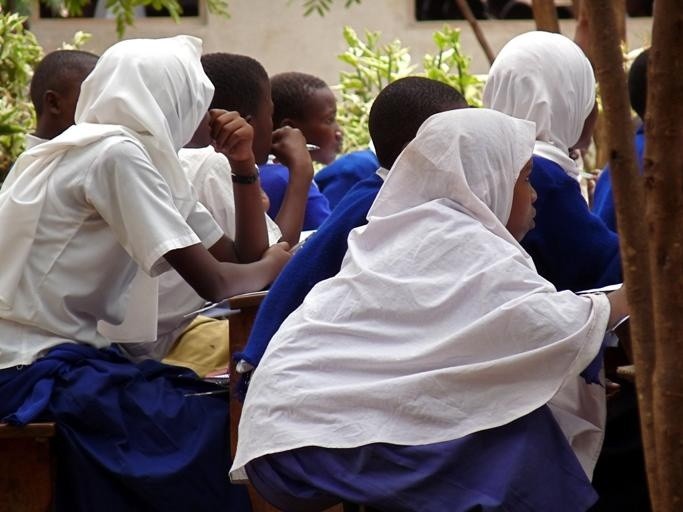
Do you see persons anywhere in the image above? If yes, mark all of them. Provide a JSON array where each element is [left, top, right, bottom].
[[1, 30, 633, 512], [594, 47, 650, 233]]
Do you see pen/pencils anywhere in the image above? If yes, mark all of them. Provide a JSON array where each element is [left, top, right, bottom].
[[288, 232, 316, 254], [305, 144, 320, 150]]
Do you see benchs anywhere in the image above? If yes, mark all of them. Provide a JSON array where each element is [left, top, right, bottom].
[[0, 420, 57, 512], [228, 289, 270, 510]]
